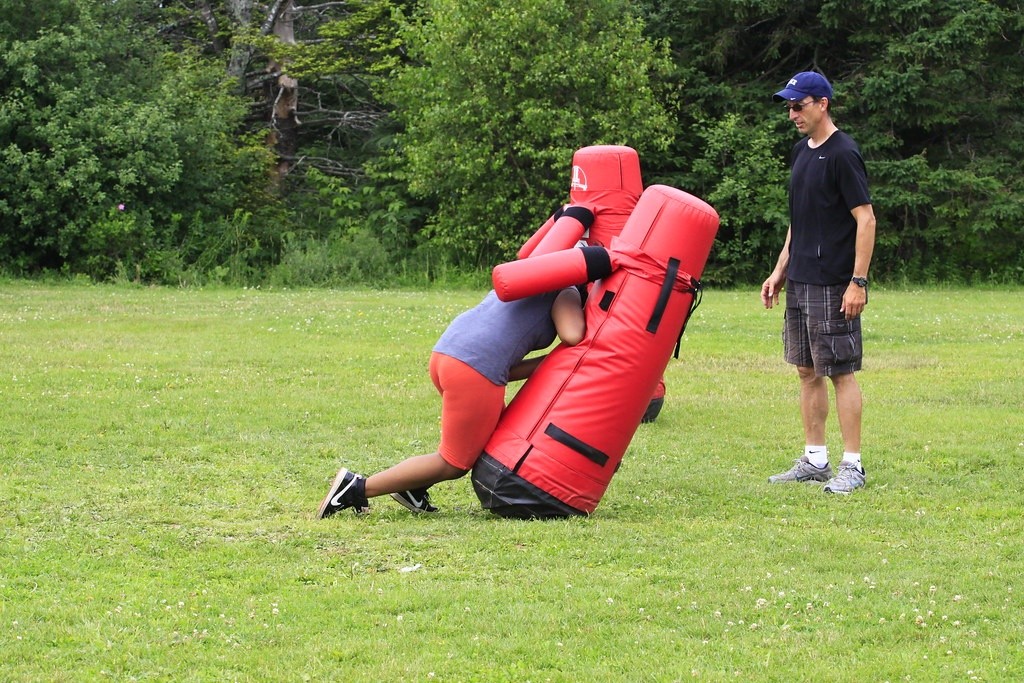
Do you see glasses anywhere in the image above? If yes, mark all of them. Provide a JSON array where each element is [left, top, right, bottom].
[[783, 100, 819, 112]]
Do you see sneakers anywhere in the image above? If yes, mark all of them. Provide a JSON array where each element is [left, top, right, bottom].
[[824, 460, 866, 495], [768, 455, 833, 484], [317, 468, 368, 520], [389, 489, 439, 515]]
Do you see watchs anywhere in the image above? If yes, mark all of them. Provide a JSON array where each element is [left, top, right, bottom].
[[852, 277, 868, 287]]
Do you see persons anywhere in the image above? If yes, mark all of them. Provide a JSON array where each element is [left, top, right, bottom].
[[319, 243, 585, 519], [760, 71, 877, 494]]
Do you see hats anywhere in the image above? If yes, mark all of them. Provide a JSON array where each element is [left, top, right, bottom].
[[772, 72, 832, 102]]
